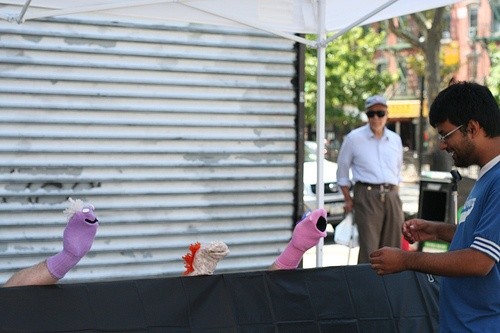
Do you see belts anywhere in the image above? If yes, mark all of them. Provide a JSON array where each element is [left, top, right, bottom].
[[356, 180, 398, 191]]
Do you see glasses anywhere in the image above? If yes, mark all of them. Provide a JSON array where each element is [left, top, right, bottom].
[[438, 124, 464, 139], [365, 110, 386, 118]]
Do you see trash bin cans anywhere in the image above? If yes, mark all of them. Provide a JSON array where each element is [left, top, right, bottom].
[[418, 178, 455, 224]]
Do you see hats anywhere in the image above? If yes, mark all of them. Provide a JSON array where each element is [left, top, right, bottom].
[[365, 95, 389, 107]]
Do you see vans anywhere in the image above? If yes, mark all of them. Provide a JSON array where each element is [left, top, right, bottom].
[[303, 141, 360, 223]]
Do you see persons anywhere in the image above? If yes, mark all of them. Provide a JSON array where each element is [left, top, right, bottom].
[[336, 95, 403, 264], [268, 208, 327, 269], [0, 207, 98, 286], [368, 81, 500, 333], [186, 241, 228, 276]]
